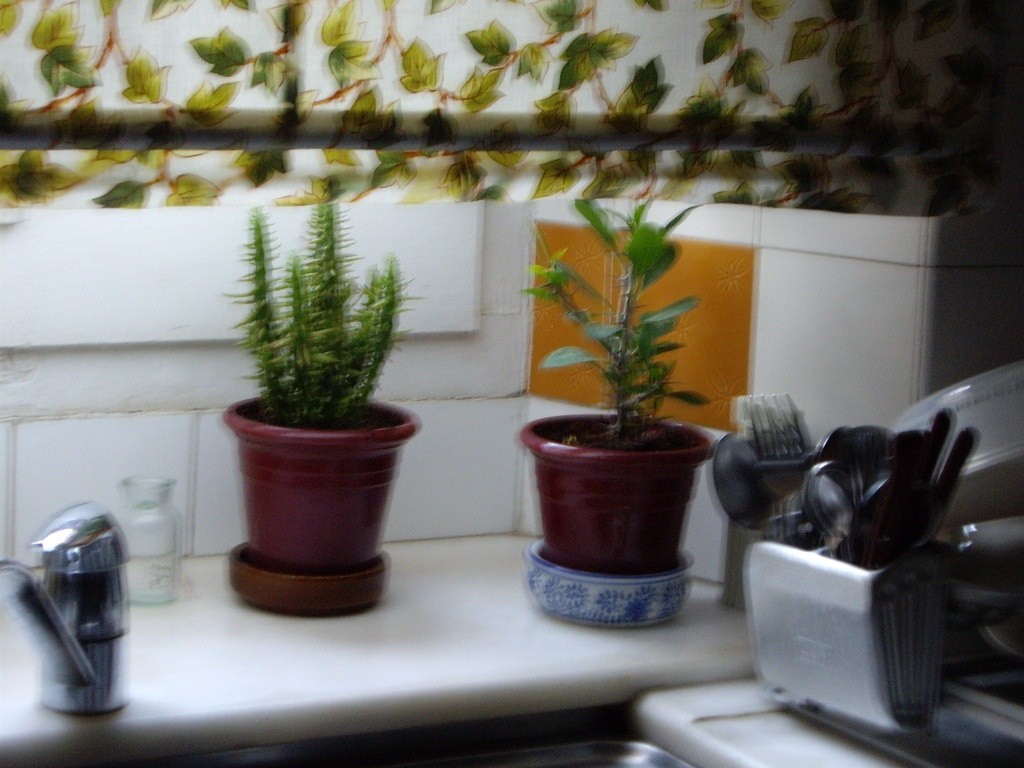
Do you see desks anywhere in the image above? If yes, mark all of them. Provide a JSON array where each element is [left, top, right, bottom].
[[636, 677, 901, 768], [0, 536, 752, 768]]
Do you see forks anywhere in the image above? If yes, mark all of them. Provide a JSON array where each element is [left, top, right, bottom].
[[737, 393, 808, 543]]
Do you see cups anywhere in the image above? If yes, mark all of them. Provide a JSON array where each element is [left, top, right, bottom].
[[720, 518, 770, 612]]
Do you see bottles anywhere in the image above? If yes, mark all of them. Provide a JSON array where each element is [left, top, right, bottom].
[[120, 477, 182, 604]]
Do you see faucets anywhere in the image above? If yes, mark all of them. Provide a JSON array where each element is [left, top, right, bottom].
[[0, 498, 133, 717]]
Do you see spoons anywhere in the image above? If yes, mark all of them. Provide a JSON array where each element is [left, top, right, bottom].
[[803, 425, 896, 569]]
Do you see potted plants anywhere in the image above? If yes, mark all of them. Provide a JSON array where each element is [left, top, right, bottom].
[[520, 182, 714, 578], [222, 178, 423, 572]]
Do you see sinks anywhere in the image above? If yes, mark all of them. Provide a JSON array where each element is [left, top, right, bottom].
[[0, 737, 701, 767]]
